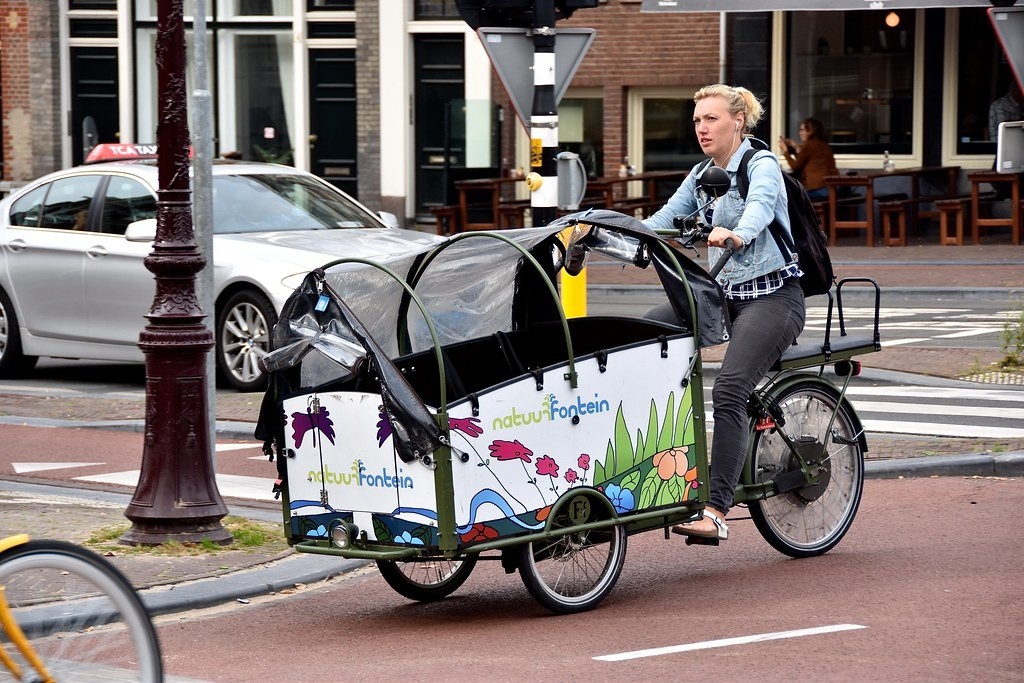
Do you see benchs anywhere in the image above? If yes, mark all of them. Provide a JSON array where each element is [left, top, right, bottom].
[[497, 199, 607, 230], [429, 198, 531, 237], [608, 200, 671, 217], [935, 192, 1007, 246], [557, 196, 650, 222], [812, 192, 907, 247], [878, 194, 967, 248]]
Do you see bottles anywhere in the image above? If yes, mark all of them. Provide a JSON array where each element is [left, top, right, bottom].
[[883, 151, 894, 172]]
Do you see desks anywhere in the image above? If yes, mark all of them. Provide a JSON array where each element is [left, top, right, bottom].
[[822, 166, 961, 248], [599, 170, 691, 220], [968, 170, 1024, 245], [454, 175, 529, 232]]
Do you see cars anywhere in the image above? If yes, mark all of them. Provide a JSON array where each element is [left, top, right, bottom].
[[0, 142, 453, 394]]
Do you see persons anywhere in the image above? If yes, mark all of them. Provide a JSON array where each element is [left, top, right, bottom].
[[779, 117, 842, 202], [989, 77, 1024, 142], [603, 82, 807, 541]]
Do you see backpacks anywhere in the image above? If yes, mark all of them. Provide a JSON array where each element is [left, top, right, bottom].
[[696, 149, 835, 299]]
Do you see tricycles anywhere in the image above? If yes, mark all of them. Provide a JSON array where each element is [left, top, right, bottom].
[[256, 166, 883, 613]]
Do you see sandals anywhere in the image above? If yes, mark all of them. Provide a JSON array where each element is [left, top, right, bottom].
[[672, 509, 730, 540]]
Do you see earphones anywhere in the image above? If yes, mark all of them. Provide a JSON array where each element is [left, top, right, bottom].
[[735, 121, 739, 131]]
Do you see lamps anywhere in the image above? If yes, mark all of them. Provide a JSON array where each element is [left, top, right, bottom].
[[885, 9, 900, 26]]
